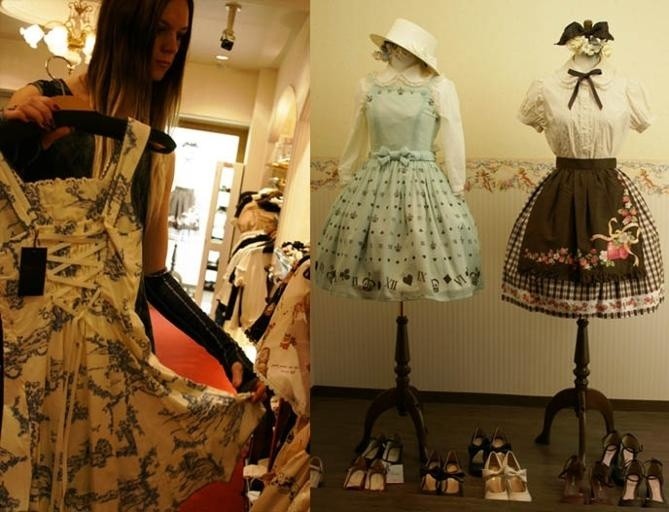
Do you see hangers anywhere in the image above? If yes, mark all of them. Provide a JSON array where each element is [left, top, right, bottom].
[[1, 56, 176, 165]]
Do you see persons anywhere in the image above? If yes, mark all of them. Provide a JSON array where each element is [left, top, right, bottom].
[[0, 0, 267, 404], [502, 19, 667, 318], [314, 19, 483, 302]]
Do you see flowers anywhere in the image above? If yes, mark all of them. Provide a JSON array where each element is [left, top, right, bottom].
[[555, 20, 613, 58]]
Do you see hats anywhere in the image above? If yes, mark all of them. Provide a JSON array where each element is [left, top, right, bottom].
[[370, 18, 441, 76]]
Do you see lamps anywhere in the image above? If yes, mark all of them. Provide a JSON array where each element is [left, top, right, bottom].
[[17, 1, 97, 67]]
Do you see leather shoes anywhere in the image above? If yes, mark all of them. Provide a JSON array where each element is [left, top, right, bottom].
[[310, 425, 666, 507]]
